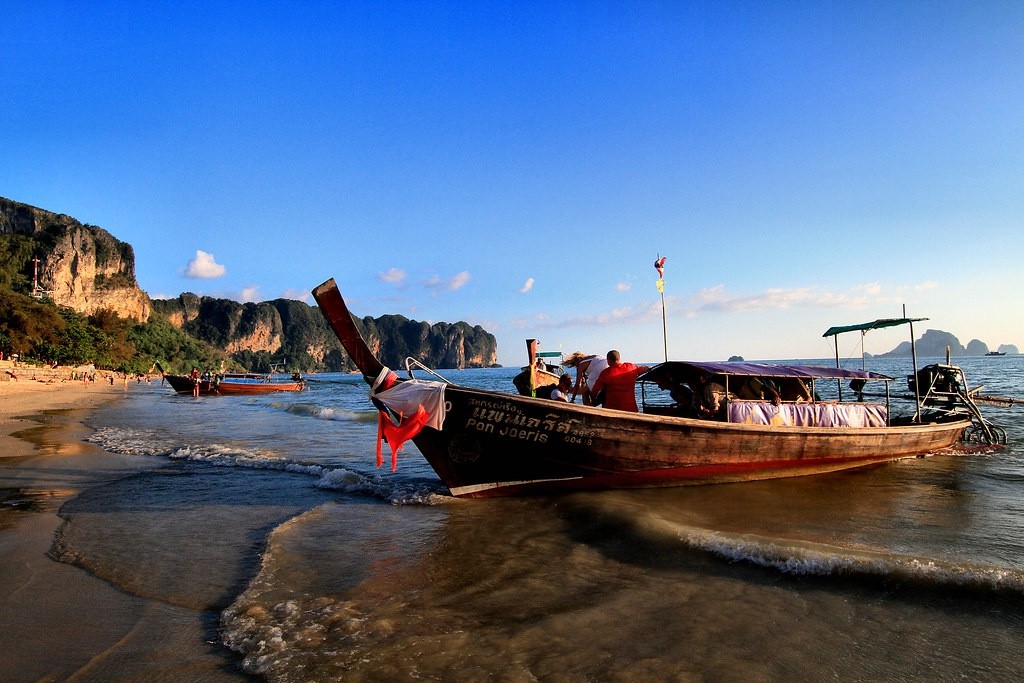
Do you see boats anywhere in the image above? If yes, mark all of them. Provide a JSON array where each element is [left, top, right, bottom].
[[312, 277, 975, 496], [985, 351, 1007, 355], [155, 361, 305, 396]]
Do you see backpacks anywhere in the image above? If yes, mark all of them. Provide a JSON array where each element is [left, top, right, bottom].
[[748, 374, 778, 400]]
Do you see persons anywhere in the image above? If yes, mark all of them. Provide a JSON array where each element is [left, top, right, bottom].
[[12, 371, 18, 382], [592, 350, 649, 413], [0, 351, 3, 360], [191, 367, 221, 397], [104, 373, 114, 386], [136, 377, 141, 385], [560, 351, 609, 408], [12, 357, 18, 367], [122, 373, 128, 387], [551, 374, 573, 403], [60, 362, 63, 366], [537, 358, 546, 371], [71, 371, 97, 383], [654, 370, 812, 421]]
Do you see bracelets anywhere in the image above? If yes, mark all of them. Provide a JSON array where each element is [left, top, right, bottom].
[[571, 398, 575, 400]]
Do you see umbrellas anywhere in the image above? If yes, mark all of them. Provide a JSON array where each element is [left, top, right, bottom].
[[12, 354, 18, 357]]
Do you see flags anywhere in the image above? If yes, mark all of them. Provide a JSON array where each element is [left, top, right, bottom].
[[536, 339, 540, 345], [654, 257, 666, 276]]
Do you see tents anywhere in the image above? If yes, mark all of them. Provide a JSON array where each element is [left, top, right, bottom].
[[73, 365, 95, 382]]
